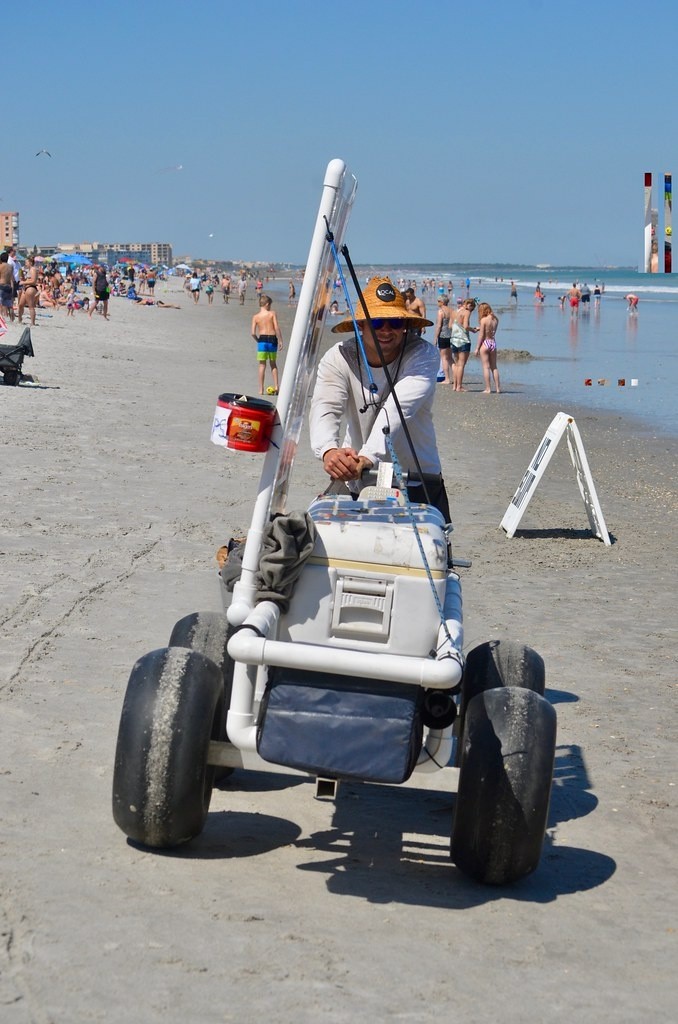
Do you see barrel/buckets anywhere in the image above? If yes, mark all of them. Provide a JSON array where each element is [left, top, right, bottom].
[[211, 393, 276, 452], [217, 570, 233, 614]]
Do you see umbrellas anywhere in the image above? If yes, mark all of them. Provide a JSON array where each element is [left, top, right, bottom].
[[0, 252, 193, 285]]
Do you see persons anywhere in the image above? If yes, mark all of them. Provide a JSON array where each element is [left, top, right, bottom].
[[173, 263, 640, 334], [433, 292, 502, 394], [0, 246, 181, 327], [309, 274, 454, 529], [250, 294, 283, 396]]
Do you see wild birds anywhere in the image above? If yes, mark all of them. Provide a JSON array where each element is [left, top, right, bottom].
[[35, 150, 52, 158]]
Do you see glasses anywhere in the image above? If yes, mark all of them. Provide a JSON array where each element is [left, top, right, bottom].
[[366, 318, 404, 330]]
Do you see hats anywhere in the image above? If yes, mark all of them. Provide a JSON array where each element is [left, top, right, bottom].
[[186, 274, 192, 277], [331, 276, 434, 332], [102, 263, 108, 269]]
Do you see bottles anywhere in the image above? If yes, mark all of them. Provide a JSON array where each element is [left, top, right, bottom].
[[19, 269, 23, 279]]
